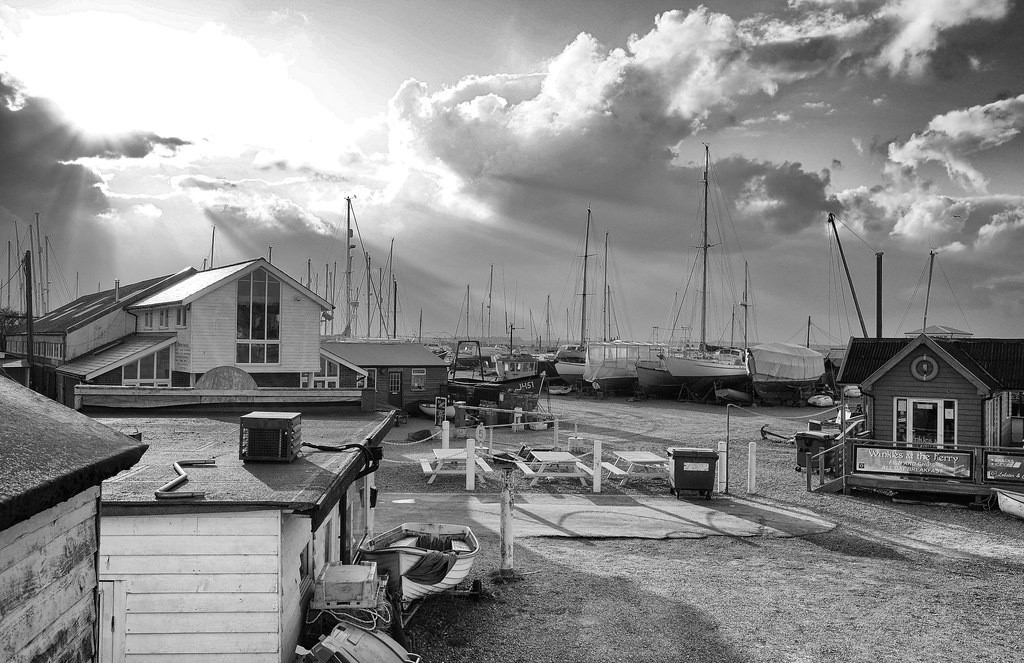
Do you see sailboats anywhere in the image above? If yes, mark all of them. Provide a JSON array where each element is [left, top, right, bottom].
[[1, 130, 975, 407]]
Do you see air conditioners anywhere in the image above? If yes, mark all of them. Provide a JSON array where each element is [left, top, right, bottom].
[[239, 410, 302, 462]]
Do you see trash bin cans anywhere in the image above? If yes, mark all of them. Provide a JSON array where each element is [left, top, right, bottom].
[[794, 429, 841, 471], [667, 447, 719, 501]]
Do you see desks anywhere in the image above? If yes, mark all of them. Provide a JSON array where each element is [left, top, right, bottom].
[[521, 451, 588, 488], [427, 449, 487, 485], [607, 451, 668, 486]]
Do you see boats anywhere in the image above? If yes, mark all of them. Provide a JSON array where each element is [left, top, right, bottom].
[[358, 521, 480, 604], [989, 487, 1024, 520], [447, 369, 546, 412]]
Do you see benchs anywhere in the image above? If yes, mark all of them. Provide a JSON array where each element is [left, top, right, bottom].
[[576, 462, 594, 476], [601, 462, 629, 476], [516, 462, 535, 477], [476, 457, 494, 474], [420, 459, 433, 476]]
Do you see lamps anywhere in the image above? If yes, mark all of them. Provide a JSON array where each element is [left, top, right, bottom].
[[923, 364, 928, 374]]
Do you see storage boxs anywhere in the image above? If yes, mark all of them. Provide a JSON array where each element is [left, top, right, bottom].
[[310, 561, 382, 608], [795, 430, 835, 474], [666, 447, 720, 499]]
